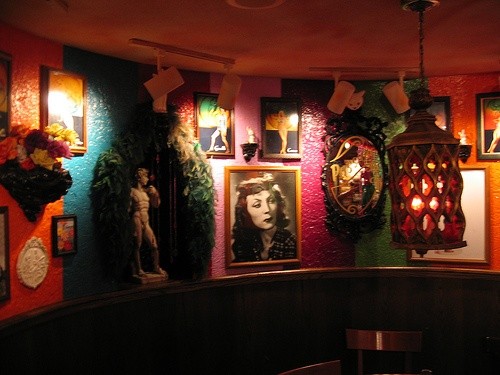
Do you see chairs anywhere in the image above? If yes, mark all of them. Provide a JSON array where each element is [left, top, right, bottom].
[[345, 327, 425, 375], [278, 359, 342, 375]]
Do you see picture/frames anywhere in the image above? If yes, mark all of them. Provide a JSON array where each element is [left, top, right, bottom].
[[51, 213, 77, 257], [405, 164, 493, 267], [1, 203, 11, 302], [258, 96, 303, 160], [222, 165, 303, 269], [191, 92, 237, 160], [406, 95, 454, 137], [90, 114, 216, 289], [0, 50, 15, 141], [39, 63, 89, 155], [475, 91, 500, 160], [322, 115, 391, 243]]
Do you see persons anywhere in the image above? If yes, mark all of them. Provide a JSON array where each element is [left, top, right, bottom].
[[127, 166, 167, 277], [231, 172, 298, 262]]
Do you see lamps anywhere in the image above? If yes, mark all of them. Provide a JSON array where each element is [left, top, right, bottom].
[[144, 55, 185, 101], [217, 63, 242, 110], [383, 72, 410, 114], [387, 87, 467, 257], [325, 71, 356, 115]]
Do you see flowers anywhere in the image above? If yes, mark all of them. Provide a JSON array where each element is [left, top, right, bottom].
[[9, 122, 85, 174]]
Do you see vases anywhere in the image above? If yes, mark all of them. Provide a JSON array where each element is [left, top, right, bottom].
[[1, 156, 73, 224]]
